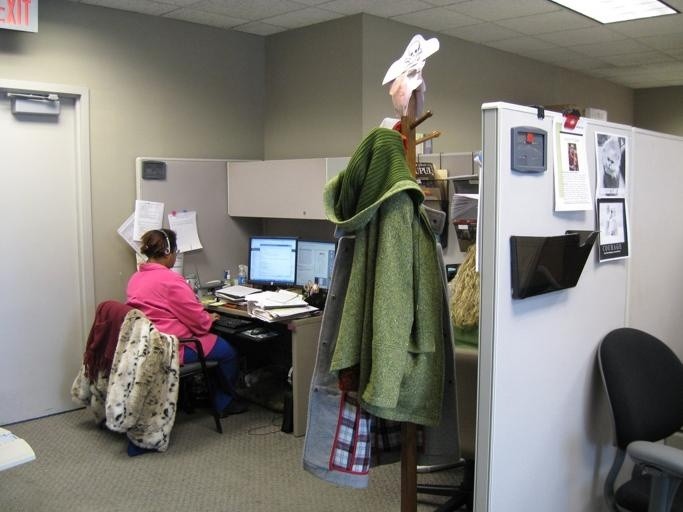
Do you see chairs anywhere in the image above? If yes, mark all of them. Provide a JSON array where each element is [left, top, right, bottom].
[[94, 301, 224, 434], [598, 327, 683, 512]]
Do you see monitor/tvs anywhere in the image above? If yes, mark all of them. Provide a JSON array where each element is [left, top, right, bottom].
[[295, 239, 338, 291], [246, 234, 298, 286]]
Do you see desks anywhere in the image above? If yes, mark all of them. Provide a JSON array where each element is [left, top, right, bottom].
[[197, 303, 326, 435]]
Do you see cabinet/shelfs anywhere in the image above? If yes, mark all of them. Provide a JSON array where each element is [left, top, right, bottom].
[[415, 153, 478, 451]]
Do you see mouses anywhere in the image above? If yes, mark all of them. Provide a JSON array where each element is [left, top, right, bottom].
[[251, 327, 267, 334]]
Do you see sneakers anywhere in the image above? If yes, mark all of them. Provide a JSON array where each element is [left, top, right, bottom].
[[218, 401, 250, 418]]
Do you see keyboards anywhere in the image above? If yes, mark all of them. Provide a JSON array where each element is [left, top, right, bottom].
[[215, 316, 252, 329]]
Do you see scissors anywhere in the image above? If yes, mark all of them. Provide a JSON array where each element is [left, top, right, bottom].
[[304, 280, 312, 293]]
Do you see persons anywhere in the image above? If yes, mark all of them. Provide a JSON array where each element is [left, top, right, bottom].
[[123, 228, 251, 419]]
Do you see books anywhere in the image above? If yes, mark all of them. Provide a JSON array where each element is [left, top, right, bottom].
[[414, 160, 448, 239], [212, 282, 320, 323], [520, 232, 582, 299], [449, 193, 478, 222]]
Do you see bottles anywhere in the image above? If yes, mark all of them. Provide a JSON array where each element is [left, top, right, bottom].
[[312, 276, 319, 293], [238, 267, 246, 286]]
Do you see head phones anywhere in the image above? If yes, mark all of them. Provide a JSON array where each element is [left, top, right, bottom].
[[144, 228, 172, 255]]
